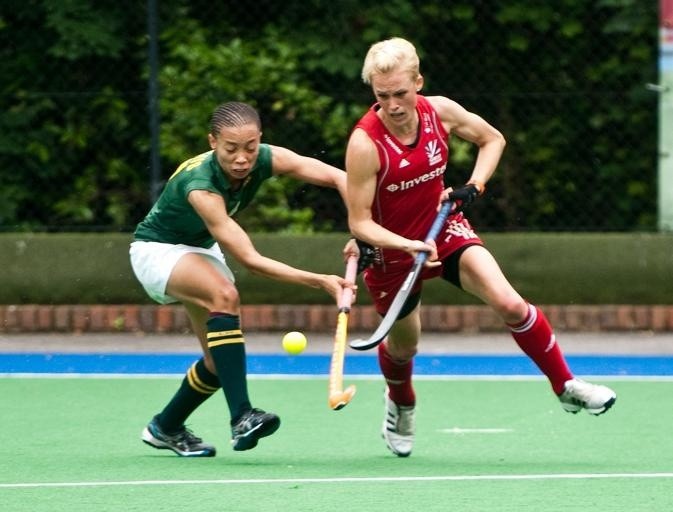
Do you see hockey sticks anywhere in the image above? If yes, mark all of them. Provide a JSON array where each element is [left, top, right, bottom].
[[349, 199, 454, 350], [327, 254, 357, 410]]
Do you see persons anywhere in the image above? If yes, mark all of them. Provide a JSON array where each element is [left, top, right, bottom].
[[129, 100, 377, 459], [343, 37, 621, 458]]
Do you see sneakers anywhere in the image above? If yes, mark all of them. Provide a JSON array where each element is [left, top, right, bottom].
[[141, 415, 216, 458], [384, 387, 414, 457], [231, 409, 280, 451], [558, 376, 617, 417]]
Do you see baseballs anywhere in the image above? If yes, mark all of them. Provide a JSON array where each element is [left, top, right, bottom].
[[283, 332, 307, 355]]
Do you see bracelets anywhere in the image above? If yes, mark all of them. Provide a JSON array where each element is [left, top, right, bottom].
[[464, 179, 486, 197]]
